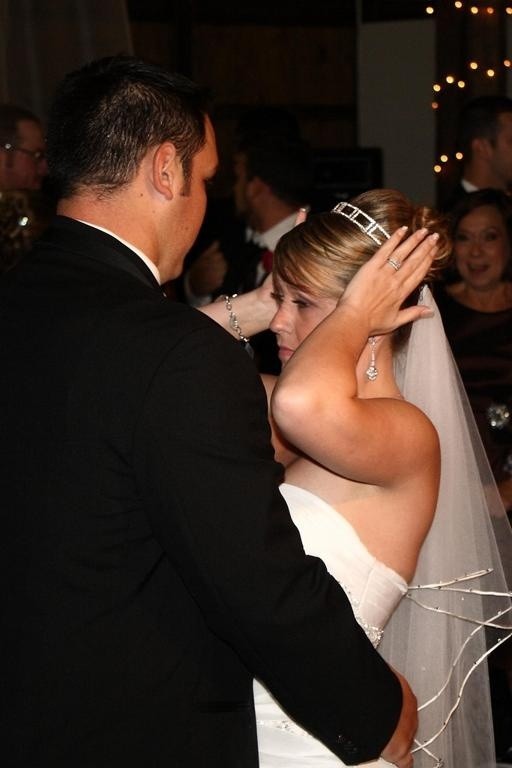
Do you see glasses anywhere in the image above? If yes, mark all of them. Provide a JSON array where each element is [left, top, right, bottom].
[[13, 143, 46, 159]]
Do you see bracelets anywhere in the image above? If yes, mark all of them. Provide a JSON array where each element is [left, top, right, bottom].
[[221, 294, 250, 344]]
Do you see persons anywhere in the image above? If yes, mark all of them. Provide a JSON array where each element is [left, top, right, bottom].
[[428, 185, 508, 535], [160, 93, 510, 316], [2, 108, 46, 229], [188, 181, 445, 768], [4, 50, 420, 768]]
